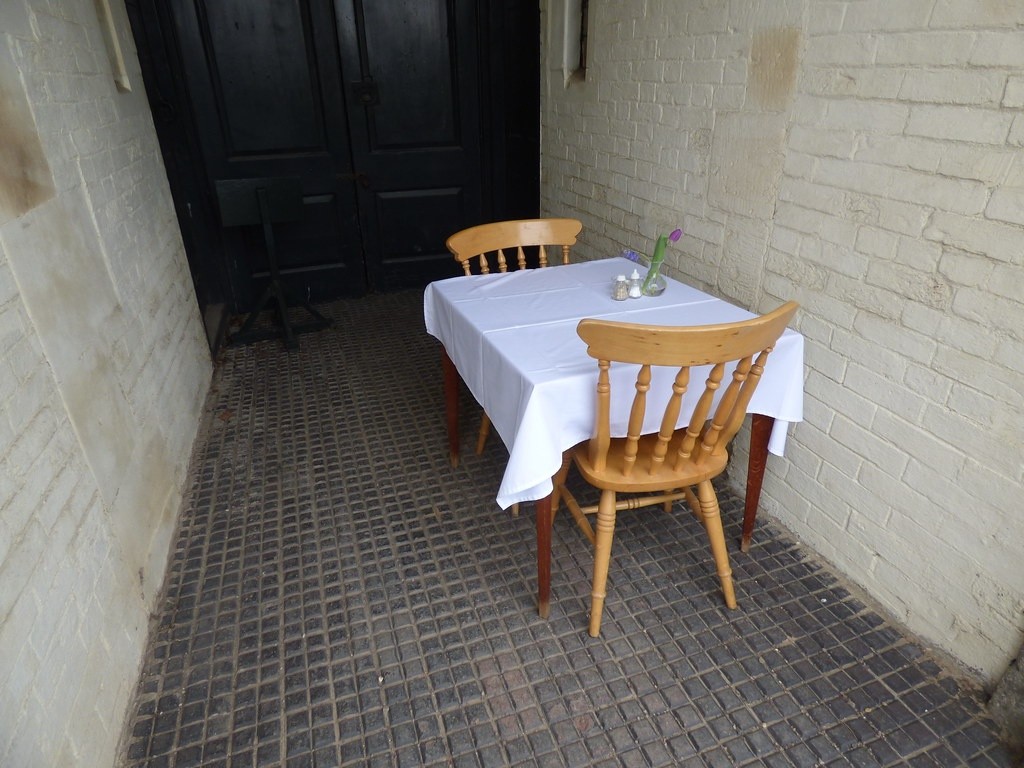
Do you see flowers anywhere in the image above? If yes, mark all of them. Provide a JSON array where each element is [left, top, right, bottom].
[[623, 229, 683, 294]]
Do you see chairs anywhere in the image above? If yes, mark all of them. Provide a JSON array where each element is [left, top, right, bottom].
[[552, 300, 800, 638], [445, 217, 585, 520]]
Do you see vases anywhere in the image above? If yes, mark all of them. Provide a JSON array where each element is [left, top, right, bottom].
[[639, 258, 667, 296]]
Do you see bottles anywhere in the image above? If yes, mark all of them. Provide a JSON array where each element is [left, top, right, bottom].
[[612, 269, 642, 301]]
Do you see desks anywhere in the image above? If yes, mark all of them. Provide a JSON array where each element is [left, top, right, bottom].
[[421, 256, 805, 618]]
